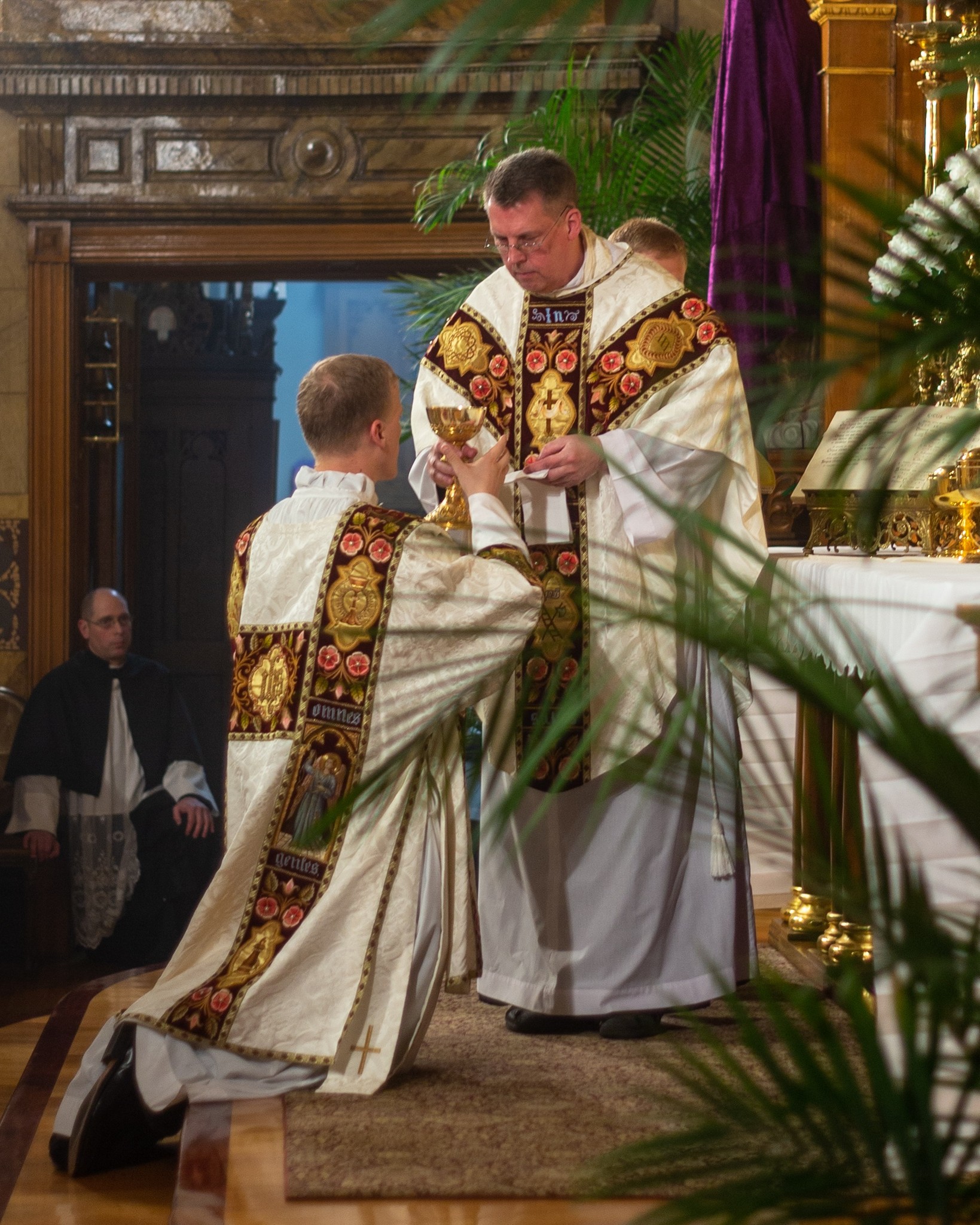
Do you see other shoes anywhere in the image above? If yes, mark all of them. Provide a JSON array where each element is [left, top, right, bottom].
[[600, 1010, 662, 1041], [505, 1005, 600, 1035]]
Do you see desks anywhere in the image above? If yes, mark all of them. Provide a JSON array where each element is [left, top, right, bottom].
[[734, 553, 980, 952]]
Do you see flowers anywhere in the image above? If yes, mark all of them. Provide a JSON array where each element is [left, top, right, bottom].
[[869, 143, 980, 303]]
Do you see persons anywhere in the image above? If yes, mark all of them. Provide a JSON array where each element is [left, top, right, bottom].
[[404, 147, 766, 1041], [48, 353, 544, 1186], [607, 218, 691, 290], [5, 586, 224, 998]]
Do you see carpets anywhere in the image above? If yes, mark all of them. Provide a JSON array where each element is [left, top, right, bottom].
[[283, 910, 907, 1202]]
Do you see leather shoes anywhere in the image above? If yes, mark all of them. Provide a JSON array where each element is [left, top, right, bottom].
[[48, 1018, 184, 1178]]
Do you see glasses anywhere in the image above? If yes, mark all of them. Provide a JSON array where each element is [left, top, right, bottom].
[[85, 613, 130, 628], [484, 205, 571, 254]]
